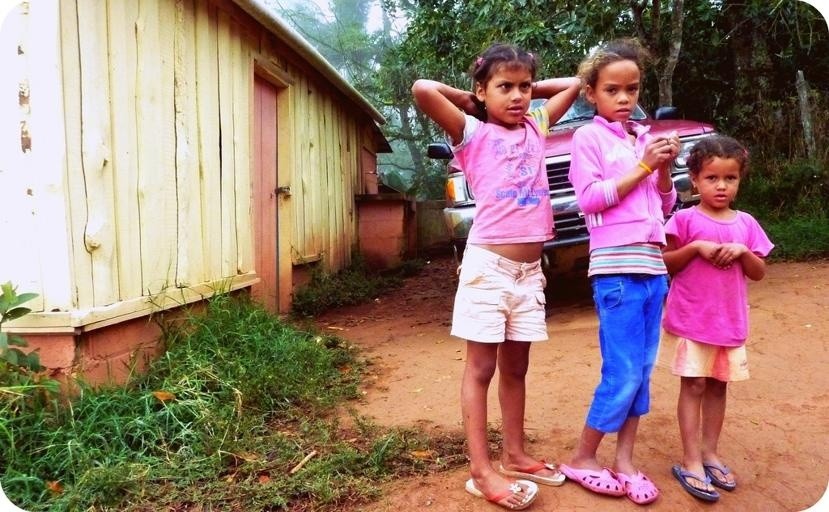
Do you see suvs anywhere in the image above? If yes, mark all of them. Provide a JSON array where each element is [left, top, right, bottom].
[[426, 83, 716, 295]]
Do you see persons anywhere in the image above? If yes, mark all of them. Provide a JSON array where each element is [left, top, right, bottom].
[[557, 36, 682, 504], [663, 136, 776, 502], [412, 44, 581, 508]]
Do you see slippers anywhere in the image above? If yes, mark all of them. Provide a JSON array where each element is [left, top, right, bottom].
[[464, 476, 540, 511], [703, 460, 736, 491], [498, 460, 566, 486], [604, 463, 659, 505], [672, 463, 720, 502], [559, 462, 625, 497]]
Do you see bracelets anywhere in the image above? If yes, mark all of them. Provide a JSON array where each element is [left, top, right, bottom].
[[638, 159, 654, 175]]
